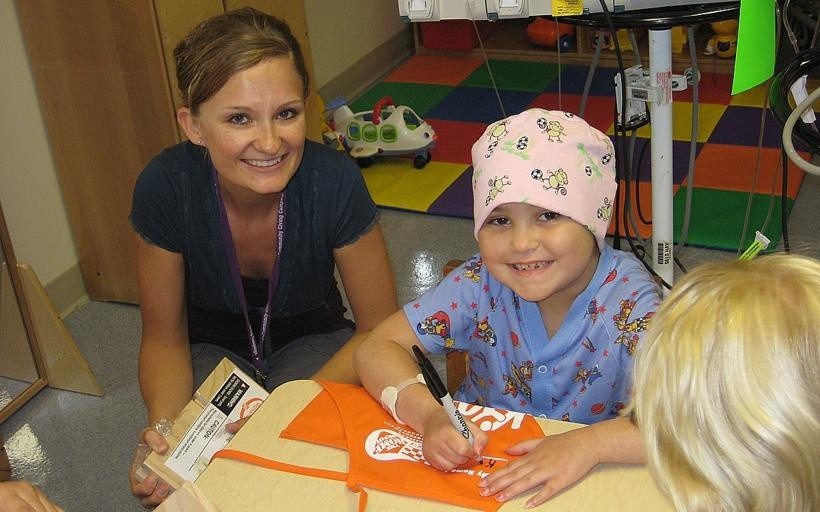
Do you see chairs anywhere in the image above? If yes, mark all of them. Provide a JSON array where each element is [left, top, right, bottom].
[[442, 260, 469, 397]]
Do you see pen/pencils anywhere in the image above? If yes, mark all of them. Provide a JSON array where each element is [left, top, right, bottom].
[[412, 345, 484, 465]]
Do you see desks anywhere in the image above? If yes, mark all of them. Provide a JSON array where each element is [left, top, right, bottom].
[[151, 379, 678, 512]]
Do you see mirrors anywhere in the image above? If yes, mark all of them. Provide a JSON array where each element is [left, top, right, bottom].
[[0, 202, 105, 426]]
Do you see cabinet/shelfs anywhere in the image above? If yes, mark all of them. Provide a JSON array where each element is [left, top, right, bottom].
[[412, 21, 735, 74]]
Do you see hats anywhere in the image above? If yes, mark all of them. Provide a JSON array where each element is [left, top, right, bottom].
[[471, 107, 618, 253]]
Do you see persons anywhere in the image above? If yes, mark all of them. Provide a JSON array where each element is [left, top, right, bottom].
[[124, 5, 404, 508], [0, 475, 65, 512], [358, 102, 668, 511], [624, 248, 820, 511]]
